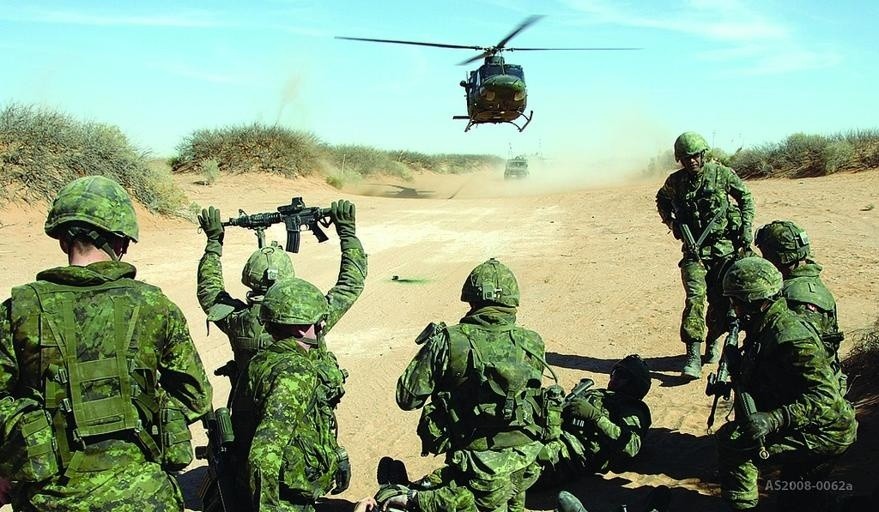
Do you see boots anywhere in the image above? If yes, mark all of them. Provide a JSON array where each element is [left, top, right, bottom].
[[682, 340, 702, 380], [705, 331, 720, 363]]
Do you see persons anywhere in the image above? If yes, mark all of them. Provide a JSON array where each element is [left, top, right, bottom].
[[705, 257, 861, 510], [377, 354, 652, 500], [1, 176, 212, 510], [196, 200, 367, 402], [755, 219, 850, 400], [394, 257, 563, 511], [354, 480, 476, 510], [228, 277, 340, 511], [655, 133, 755, 379]]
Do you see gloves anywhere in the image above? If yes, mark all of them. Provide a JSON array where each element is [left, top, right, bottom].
[[738, 223, 753, 248], [197, 205, 225, 256], [570, 398, 602, 420], [331, 199, 364, 251], [742, 410, 785, 441], [332, 459, 351, 495]]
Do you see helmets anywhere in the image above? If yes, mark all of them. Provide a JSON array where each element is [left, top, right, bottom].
[[460, 259, 520, 306], [44, 175, 138, 243], [675, 131, 711, 163], [259, 277, 330, 325], [241, 246, 295, 292], [723, 257, 784, 301], [610, 354, 651, 389], [759, 220, 811, 264]]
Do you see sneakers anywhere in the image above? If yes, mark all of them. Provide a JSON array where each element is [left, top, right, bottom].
[[378, 457, 391, 489], [393, 461, 408, 488]]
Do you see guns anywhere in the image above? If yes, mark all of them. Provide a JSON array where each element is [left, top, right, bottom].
[[671, 200, 704, 267], [722, 345, 770, 460], [196, 360, 235, 512], [706, 304, 741, 435], [198, 197, 332, 253]]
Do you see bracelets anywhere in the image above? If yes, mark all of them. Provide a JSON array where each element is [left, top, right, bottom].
[[406, 488, 420, 510]]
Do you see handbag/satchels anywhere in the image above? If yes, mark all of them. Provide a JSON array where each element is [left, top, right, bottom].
[[159, 396, 195, 470], [0, 397, 59, 485]]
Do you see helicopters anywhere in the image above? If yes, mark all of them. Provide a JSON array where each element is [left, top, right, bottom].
[[335, 14, 643, 134]]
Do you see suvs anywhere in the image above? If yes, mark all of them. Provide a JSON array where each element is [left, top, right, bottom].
[[502, 156, 529, 179]]
